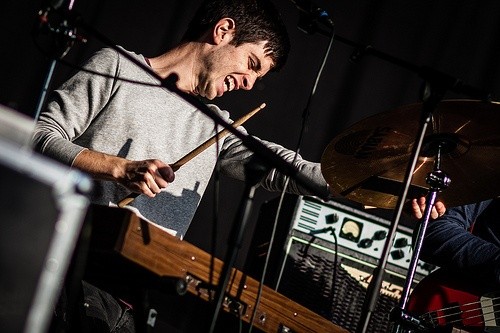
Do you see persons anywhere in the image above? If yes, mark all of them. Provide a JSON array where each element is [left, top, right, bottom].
[[416, 162, 500, 290], [34, 1, 445, 333]]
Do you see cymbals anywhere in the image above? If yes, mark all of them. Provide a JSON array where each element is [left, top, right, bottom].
[[320, 99, 500, 210]]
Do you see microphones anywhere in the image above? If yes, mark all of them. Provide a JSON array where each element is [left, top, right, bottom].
[[290, 0, 335, 28]]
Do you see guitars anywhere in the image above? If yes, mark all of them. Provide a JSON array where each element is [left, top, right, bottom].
[[403, 255, 499, 333]]
